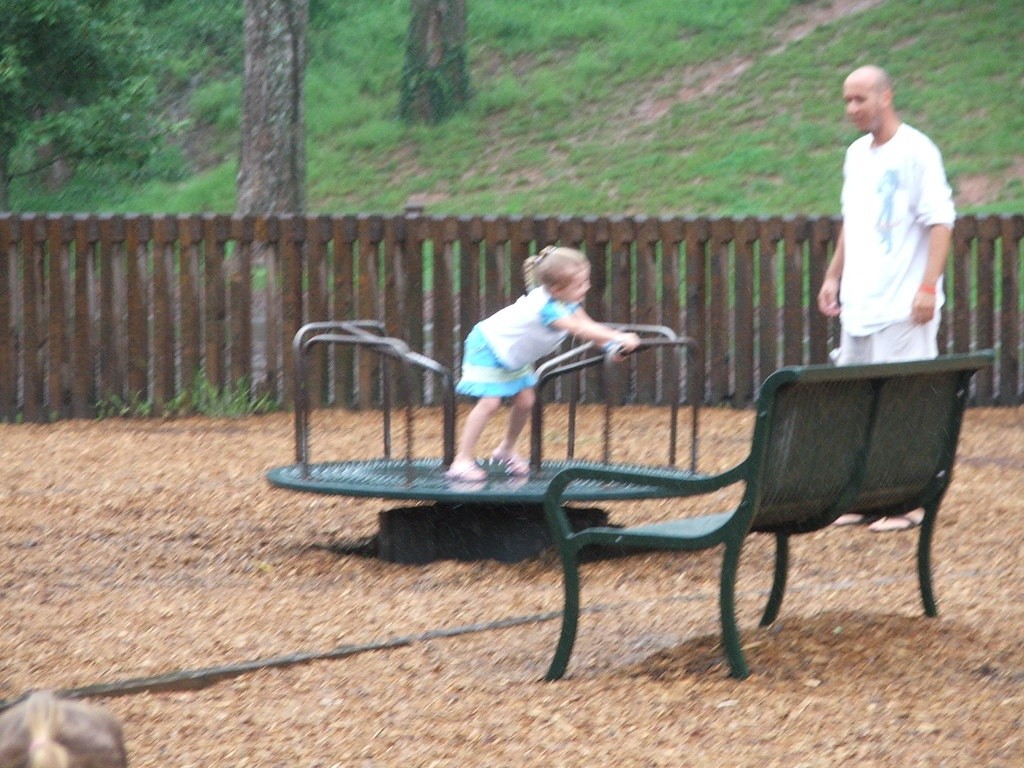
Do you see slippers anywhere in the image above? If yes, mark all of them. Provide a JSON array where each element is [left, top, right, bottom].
[[872, 514, 922, 532], [834, 512, 878, 526]]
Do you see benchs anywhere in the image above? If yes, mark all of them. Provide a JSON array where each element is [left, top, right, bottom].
[[544, 349, 997, 689]]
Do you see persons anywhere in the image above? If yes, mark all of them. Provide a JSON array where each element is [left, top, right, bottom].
[[817, 65, 957, 532], [446, 246, 641, 483]]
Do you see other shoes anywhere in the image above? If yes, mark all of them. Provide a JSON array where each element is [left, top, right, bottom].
[[493, 449, 528, 476], [449, 457, 486, 480]]
